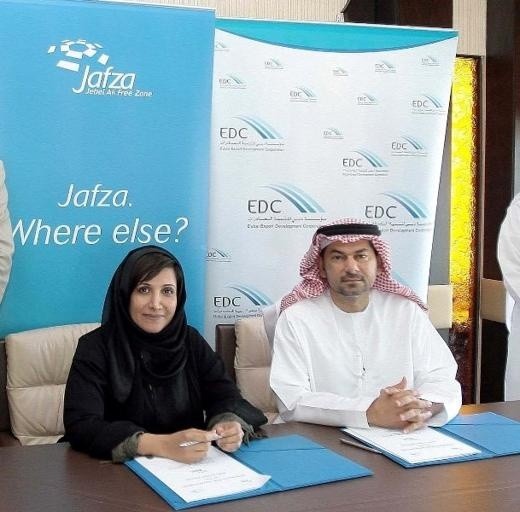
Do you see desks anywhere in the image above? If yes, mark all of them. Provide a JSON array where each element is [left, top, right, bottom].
[[0, 400, 518, 511]]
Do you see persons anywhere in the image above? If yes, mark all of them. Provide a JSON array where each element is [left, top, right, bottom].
[[0, 160, 16, 304], [62, 244, 270, 466], [270, 216, 464, 433], [495, 191, 520, 402]]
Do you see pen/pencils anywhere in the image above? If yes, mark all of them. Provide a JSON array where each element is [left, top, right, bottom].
[[178, 436, 220, 448], [340, 439, 383, 455]]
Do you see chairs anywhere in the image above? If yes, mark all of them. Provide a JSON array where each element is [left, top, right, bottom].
[[214, 315, 276, 416], [0, 323, 102, 445]]
